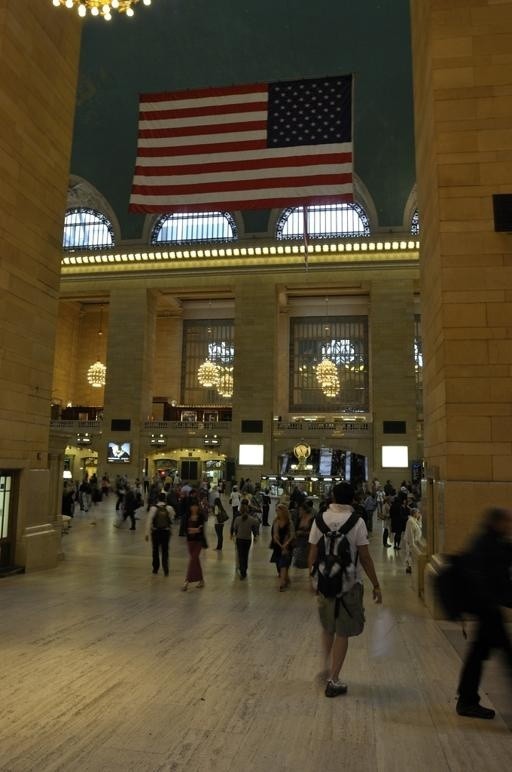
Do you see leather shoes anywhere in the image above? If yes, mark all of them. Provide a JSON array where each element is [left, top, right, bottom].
[[280, 578, 292, 593], [196, 582, 205, 588], [180, 587, 188, 591]]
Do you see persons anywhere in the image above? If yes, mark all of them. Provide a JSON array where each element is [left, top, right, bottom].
[[353, 477, 423, 575], [108, 442, 130, 458], [64, 471, 315, 592], [456, 505, 512, 718], [307, 483, 383, 698]]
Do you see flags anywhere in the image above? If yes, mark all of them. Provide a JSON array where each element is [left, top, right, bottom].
[[128, 73, 354, 214]]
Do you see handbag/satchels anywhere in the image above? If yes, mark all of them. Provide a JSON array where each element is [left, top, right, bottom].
[[216, 509, 229, 524]]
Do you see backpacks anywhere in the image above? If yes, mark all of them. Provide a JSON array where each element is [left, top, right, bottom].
[[431, 555, 475, 624], [309, 510, 360, 598], [152, 502, 172, 530]]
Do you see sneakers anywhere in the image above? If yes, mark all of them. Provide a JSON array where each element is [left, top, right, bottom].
[[323, 679, 352, 700], [456, 699, 497, 721]]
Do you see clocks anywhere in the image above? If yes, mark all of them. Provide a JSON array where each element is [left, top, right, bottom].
[[293, 441, 312, 460]]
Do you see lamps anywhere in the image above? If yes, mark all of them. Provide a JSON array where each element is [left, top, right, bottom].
[[322, 377, 341, 398], [217, 372, 234, 399], [315, 297, 338, 389], [85, 302, 107, 389], [196, 300, 220, 389]]
